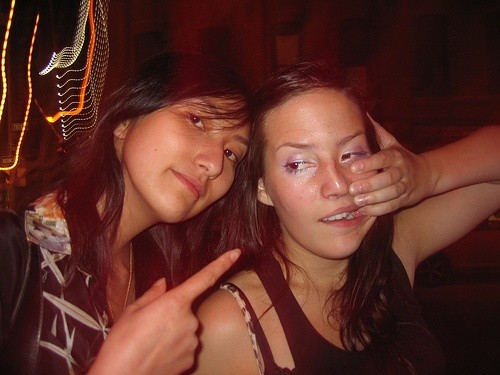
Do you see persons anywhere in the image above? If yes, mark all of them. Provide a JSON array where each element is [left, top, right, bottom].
[[196, 62, 500, 375], [0, 56, 252, 375]]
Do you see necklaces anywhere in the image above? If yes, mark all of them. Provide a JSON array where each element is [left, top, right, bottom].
[[106, 241, 132, 323]]
